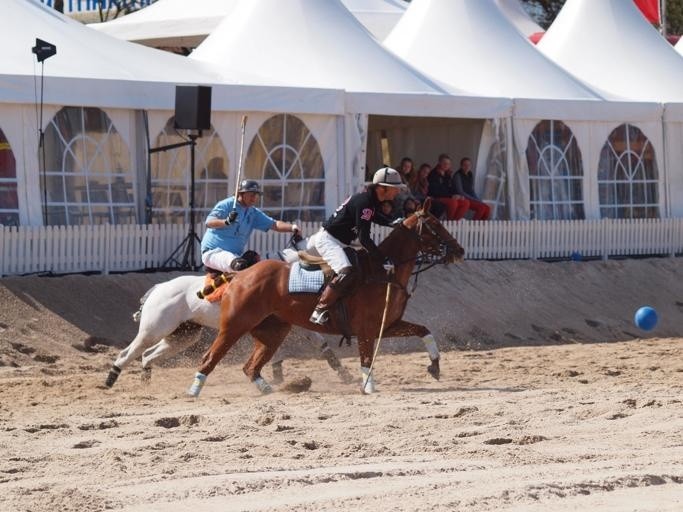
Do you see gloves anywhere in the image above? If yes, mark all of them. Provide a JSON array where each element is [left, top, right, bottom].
[[292, 224, 302, 237], [225, 211, 238, 226], [382, 256, 395, 275]]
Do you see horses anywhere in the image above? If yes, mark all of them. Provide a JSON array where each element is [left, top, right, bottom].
[[184, 196, 465, 397], [99, 238, 369, 395]]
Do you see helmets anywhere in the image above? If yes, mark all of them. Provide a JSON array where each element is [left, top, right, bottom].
[[373, 166, 408, 188], [238, 179, 264, 195]]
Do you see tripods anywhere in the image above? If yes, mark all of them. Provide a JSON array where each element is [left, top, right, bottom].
[[159, 129, 203, 271]]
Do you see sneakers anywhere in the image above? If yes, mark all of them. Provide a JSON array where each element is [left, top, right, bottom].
[[309, 308, 329, 325]]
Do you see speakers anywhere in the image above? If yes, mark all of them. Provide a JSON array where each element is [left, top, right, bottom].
[[174, 86, 211, 129]]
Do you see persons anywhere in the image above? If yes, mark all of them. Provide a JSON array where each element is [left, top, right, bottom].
[[427, 154, 470, 221], [416, 163, 447, 221], [400, 197, 417, 219], [396, 157, 422, 211], [379, 200, 403, 221], [308, 166, 406, 327], [452, 157, 490, 220], [199, 178, 302, 273]]
